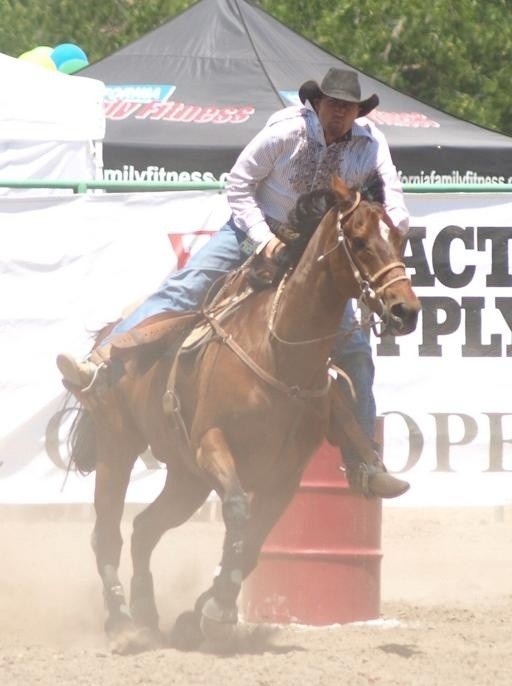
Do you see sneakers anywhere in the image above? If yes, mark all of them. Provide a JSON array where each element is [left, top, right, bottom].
[[56, 353, 107, 405], [348, 461, 410, 500]]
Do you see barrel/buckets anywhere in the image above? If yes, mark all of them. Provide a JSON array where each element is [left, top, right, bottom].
[[236, 414, 382, 625]]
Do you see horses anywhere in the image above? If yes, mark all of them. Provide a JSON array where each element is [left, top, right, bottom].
[[53, 167, 420, 655]]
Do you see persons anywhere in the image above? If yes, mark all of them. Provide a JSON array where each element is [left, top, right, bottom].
[[55, 69, 410, 500]]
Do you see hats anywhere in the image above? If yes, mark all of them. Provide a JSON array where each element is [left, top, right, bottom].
[[299, 67, 379, 118]]
